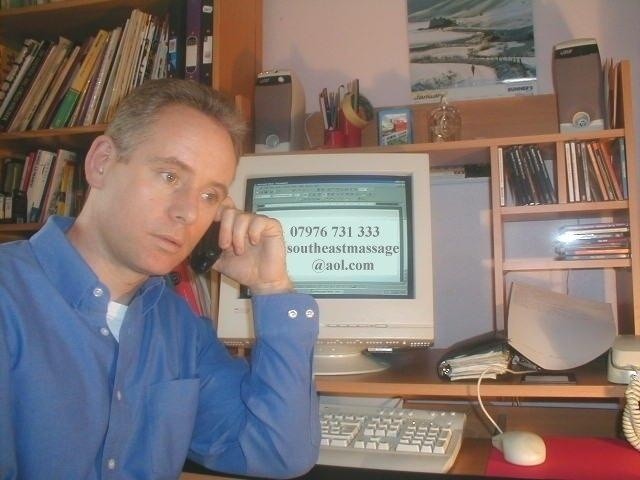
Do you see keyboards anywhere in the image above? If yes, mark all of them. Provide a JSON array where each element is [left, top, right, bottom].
[[316, 404, 466, 474]]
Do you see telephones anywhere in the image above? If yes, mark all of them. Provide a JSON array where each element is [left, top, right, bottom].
[[607, 335, 640, 384]]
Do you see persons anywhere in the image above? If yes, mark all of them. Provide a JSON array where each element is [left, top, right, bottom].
[[471, 63, 475, 76], [1, 78, 323, 477]]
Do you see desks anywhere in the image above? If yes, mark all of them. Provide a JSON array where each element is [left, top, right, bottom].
[[179, 404, 639, 479]]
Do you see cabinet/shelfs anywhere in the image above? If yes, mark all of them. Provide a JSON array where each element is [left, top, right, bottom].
[[1, 0, 264, 356], [230, 57, 640, 398]]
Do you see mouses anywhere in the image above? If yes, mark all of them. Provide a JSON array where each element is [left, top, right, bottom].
[[492, 430, 547, 466]]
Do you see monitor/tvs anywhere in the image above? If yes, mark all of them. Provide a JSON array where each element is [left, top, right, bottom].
[[216, 153, 435, 375]]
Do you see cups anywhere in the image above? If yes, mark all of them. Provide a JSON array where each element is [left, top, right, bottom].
[[323, 129, 344, 147], [341, 106, 362, 147]]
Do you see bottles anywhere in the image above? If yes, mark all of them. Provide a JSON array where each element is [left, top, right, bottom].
[[425, 92, 462, 144]]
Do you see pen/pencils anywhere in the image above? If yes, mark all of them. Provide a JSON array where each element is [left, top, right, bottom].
[[320, 79, 359, 131]]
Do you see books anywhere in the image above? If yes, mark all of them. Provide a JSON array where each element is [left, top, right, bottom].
[[498, 60, 628, 259], [436, 330, 507, 382]]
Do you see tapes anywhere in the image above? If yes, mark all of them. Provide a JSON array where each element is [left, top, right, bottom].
[[343, 94, 375, 128]]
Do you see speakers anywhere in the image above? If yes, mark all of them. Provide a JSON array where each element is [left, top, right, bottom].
[[554, 38, 606, 134], [254, 69, 305, 154]]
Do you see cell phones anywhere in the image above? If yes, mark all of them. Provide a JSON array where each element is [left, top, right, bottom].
[[190, 221, 223, 273]]
